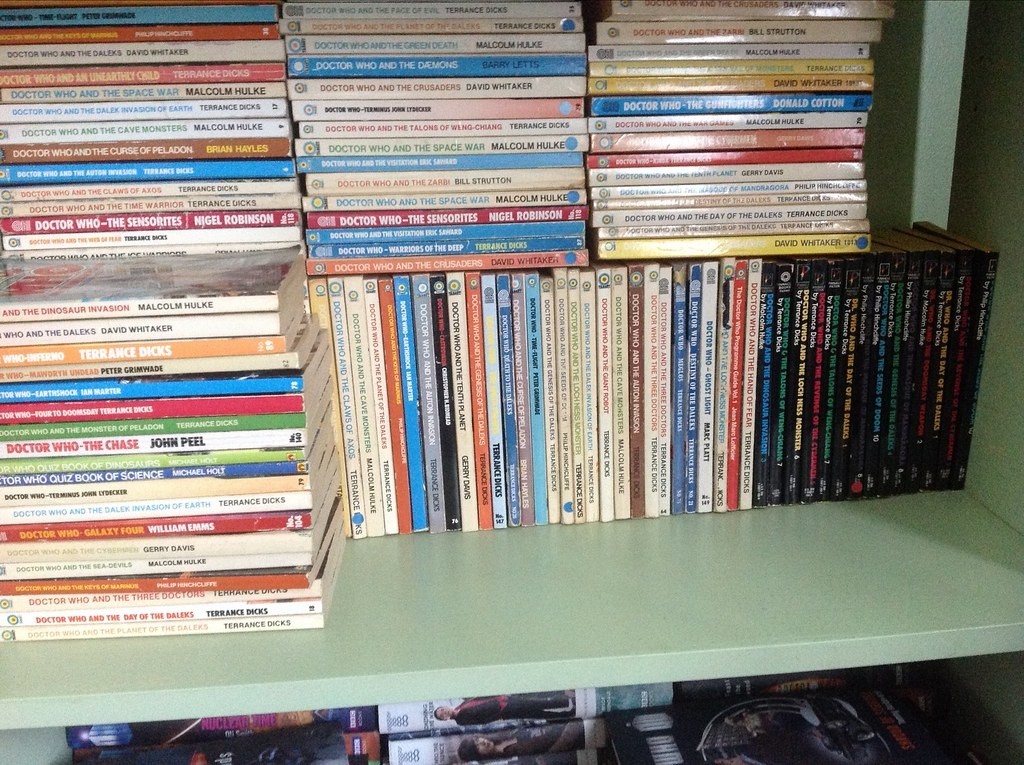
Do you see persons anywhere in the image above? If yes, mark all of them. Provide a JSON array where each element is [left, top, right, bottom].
[[456, 720, 586, 763], [434, 690, 578, 727]]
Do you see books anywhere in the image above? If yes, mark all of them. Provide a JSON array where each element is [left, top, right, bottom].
[[0, 0, 999, 643], [66, 660, 988, 765]]
